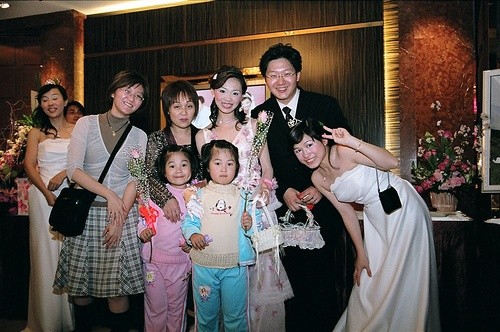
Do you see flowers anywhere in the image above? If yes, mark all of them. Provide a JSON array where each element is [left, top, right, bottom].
[[244, 110, 270, 234], [129, 149, 154, 263], [407, 99, 491, 194], [0, 114, 36, 184]]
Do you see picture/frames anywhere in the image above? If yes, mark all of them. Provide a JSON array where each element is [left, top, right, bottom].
[[159, 67, 271, 129]]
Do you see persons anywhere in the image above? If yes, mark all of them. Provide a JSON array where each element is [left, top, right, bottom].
[[64, 100, 85, 124], [195, 66, 294, 332], [181, 140, 256, 332], [145, 78, 203, 224], [53, 70, 149, 332], [249, 43, 352, 332], [136, 145, 200, 332], [286, 116, 442, 332], [20, 79, 75, 332]]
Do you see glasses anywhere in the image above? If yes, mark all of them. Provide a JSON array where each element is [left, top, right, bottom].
[[118, 87, 145, 102], [264, 71, 297, 79]]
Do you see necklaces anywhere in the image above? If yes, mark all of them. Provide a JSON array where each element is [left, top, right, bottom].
[[323, 177, 327, 181], [218, 118, 235, 124], [106, 113, 129, 136]]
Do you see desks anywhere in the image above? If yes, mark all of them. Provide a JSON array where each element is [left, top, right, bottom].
[[354, 217, 500, 332]]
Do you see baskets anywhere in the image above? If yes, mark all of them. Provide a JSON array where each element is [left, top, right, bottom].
[[277, 203, 324, 250], [251, 197, 286, 253]]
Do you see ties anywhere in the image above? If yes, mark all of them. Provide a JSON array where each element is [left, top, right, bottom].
[[282, 106, 294, 125]]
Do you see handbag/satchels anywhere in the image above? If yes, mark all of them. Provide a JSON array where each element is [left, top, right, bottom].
[[48, 186, 96, 237], [378, 186, 402, 215]]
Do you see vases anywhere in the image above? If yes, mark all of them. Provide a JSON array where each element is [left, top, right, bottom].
[[430, 192, 458, 213]]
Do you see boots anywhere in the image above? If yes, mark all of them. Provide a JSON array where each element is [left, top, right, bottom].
[[73, 302, 93, 332], [110, 308, 130, 332]]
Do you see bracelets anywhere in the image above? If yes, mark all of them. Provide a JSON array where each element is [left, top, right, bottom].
[[355, 139, 363, 152]]
